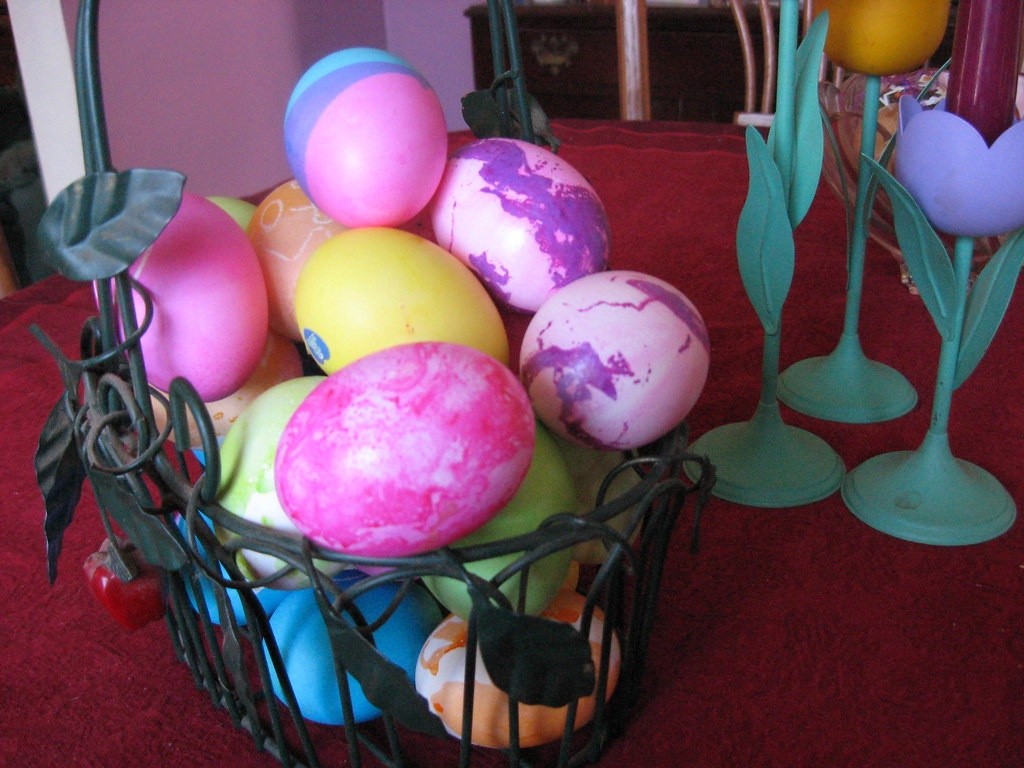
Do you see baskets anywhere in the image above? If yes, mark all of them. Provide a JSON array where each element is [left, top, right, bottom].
[[31, 0, 717, 768]]
